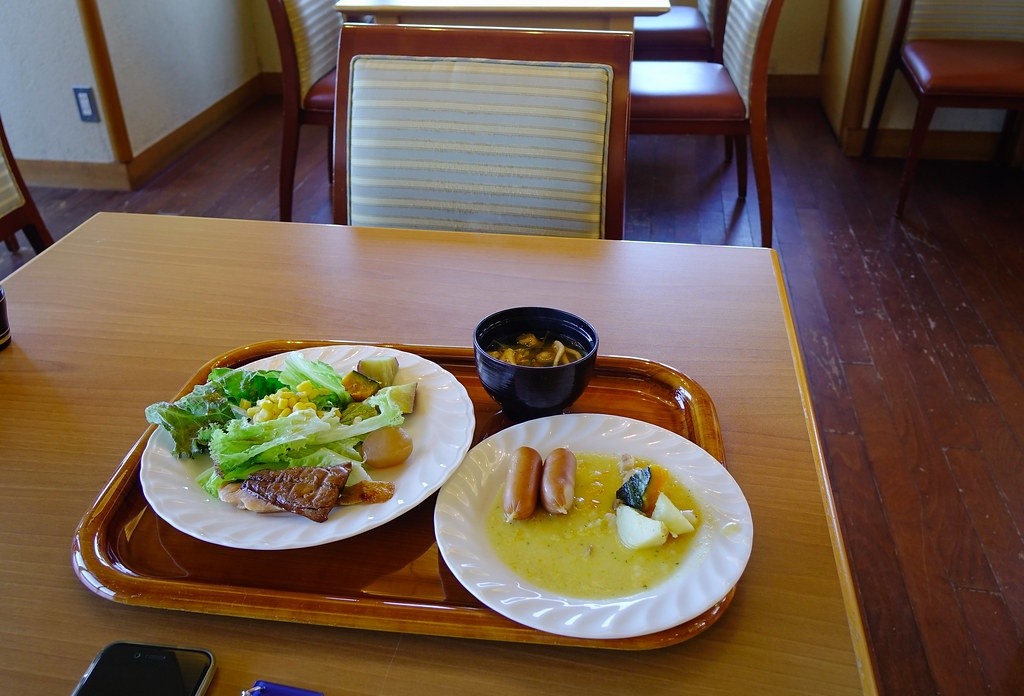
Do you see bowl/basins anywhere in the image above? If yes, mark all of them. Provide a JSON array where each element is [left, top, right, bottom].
[[472, 307, 599, 421]]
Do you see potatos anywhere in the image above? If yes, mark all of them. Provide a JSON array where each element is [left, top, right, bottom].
[[615, 493, 696, 549]]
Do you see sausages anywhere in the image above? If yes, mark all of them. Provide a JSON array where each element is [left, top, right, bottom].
[[502, 445, 577, 521]]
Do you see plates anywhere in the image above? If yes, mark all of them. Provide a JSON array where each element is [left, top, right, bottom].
[[433, 413, 754, 639], [140, 345, 477, 551]]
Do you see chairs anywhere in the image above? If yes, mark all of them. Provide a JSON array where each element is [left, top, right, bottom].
[[333, 21, 632, 245], [268, 0, 352, 221], [0, 119, 55, 258], [862, 0, 1024, 225], [624, 0, 784, 246]]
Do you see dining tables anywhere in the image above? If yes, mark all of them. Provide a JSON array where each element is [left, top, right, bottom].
[[0, 211, 884, 696]]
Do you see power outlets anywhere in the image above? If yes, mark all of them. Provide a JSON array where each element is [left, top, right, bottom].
[[73, 87, 100, 123]]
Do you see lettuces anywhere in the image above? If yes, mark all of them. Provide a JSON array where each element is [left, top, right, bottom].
[[145, 352, 404, 486]]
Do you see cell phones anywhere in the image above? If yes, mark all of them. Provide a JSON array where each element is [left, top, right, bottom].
[[71, 641, 217, 696]]
